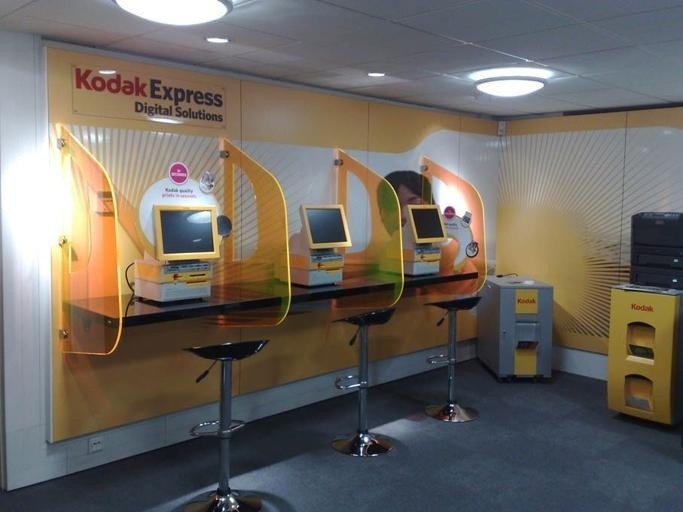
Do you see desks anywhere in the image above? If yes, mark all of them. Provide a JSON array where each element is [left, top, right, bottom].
[[63, 270, 479, 328]]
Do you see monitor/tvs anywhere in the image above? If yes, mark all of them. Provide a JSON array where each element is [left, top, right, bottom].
[[299, 204, 352, 249], [406, 204, 449, 243], [153, 204, 220, 261]]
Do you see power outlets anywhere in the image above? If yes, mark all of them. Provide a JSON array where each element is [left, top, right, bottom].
[[87, 437, 103, 454]]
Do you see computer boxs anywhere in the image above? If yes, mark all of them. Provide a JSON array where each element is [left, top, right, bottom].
[[275, 253, 344, 286], [134, 260, 211, 302], [379, 247, 441, 275]]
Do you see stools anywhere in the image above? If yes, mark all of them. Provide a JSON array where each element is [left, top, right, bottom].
[[182, 339, 272, 512], [423, 297, 482, 422], [331, 308, 395, 457]]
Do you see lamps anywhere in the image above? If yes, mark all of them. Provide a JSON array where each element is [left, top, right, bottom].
[[112, 0, 234, 25], [473, 76, 547, 97]]
